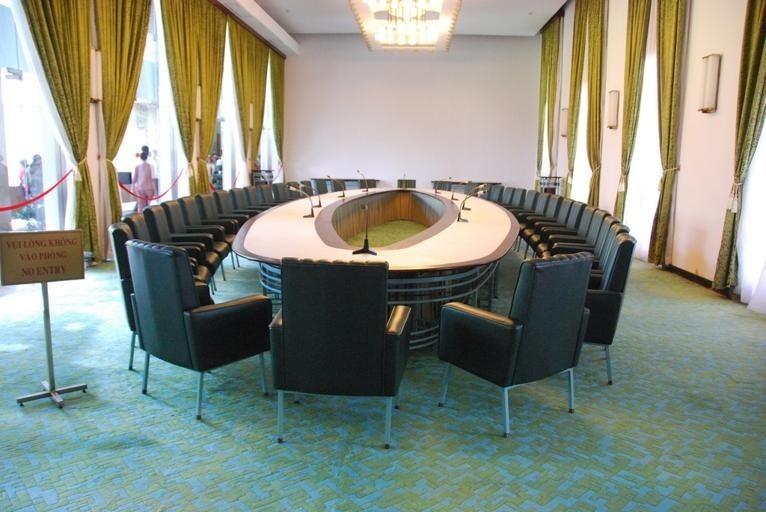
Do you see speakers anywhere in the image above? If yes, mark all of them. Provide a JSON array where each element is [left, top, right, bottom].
[[252, 170, 272, 186]]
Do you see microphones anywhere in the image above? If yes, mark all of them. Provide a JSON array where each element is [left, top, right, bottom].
[[326, 175, 345, 197], [435, 176, 452, 193], [352, 203, 377, 256], [356, 169, 368, 192], [462, 184, 488, 210], [297, 183, 322, 207], [455, 189, 490, 223], [288, 186, 315, 218], [451, 179, 472, 201]]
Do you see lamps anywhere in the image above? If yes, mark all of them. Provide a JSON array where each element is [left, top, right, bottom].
[[560, 108, 568, 137], [605, 90, 620, 130], [194, 83, 203, 120], [249, 103, 255, 130], [696, 53, 723, 113], [348, 0, 463, 55], [87, 47, 104, 103]]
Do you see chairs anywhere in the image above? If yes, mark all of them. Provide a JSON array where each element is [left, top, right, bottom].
[[243, 177, 380, 225], [432, 181, 451, 191], [435, 252, 593, 438], [574, 200, 638, 386], [267, 257, 412, 451], [397, 178, 416, 188], [122, 187, 242, 296], [124, 238, 273, 423], [108, 221, 216, 372], [462, 182, 573, 259]]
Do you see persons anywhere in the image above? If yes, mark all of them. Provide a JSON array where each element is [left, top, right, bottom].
[[205, 154, 211, 164], [132, 153, 157, 215], [19, 159, 32, 200], [24, 155, 45, 230], [135, 147, 152, 211], [206, 153, 218, 191]]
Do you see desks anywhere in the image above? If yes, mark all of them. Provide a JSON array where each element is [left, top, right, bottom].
[[231, 186, 521, 352]]
